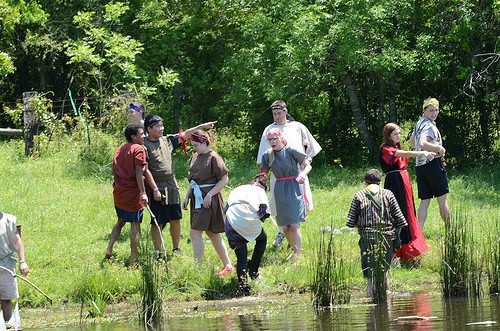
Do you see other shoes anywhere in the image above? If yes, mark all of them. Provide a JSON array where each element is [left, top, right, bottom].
[[272, 245, 284, 252], [219, 268, 232, 275], [157, 252, 170, 261], [172, 248, 180, 255], [250, 272, 260, 280]]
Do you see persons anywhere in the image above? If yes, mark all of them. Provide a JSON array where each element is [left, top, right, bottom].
[[129, 99, 146, 126], [256, 100, 322, 249], [142, 114, 218, 261], [378, 123, 437, 262], [106, 123, 148, 268], [262, 126, 313, 264], [0, 211, 30, 331], [346, 168, 408, 296], [182, 130, 233, 277], [408, 97, 451, 231], [225, 172, 270, 296]]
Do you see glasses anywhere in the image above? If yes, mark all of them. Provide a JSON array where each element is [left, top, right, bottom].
[[267, 137, 278, 141]]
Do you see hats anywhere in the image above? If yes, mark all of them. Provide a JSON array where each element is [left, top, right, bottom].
[[423, 98, 439, 110], [365, 169, 382, 184]]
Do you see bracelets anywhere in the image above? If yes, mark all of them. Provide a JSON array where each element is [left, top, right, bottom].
[[439, 146, 443, 151], [20, 260, 24, 263], [140, 192, 146, 194]]
